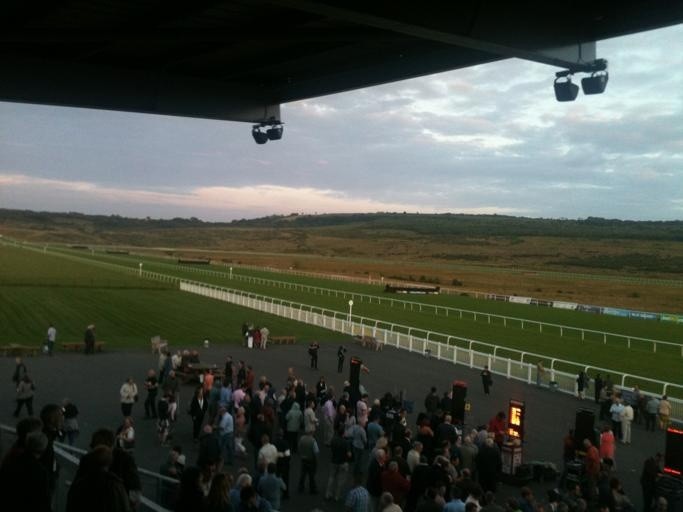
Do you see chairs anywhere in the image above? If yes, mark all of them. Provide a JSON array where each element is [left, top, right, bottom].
[[151, 335, 168, 354]]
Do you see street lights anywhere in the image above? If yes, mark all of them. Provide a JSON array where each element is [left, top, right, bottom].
[[229, 267, 233, 280], [348, 298, 354, 325], [138, 263, 144, 276]]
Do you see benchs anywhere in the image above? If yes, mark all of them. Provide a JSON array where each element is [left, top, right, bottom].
[[270, 337, 296, 345], [621, 390, 661, 424], [0, 346, 40, 356], [64, 342, 105, 351], [352, 336, 384, 352]]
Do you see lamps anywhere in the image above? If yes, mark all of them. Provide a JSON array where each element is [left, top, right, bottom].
[[553, 59, 609, 103], [252, 120, 284, 144]]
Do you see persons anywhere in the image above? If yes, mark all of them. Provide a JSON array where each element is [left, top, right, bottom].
[[46, 324, 55, 358], [285, 368, 296, 383], [157, 348, 200, 385], [335, 345, 346, 373], [83, 324, 95, 354], [1, 371, 672, 512], [245, 366, 254, 397], [536, 358, 544, 388], [11, 355, 27, 388], [307, 340, 319, 370], [236, 359, 245, 387], [478, 365, 492, 396], [223, 354, 235, 387], [240, 321, 269, 349]]
